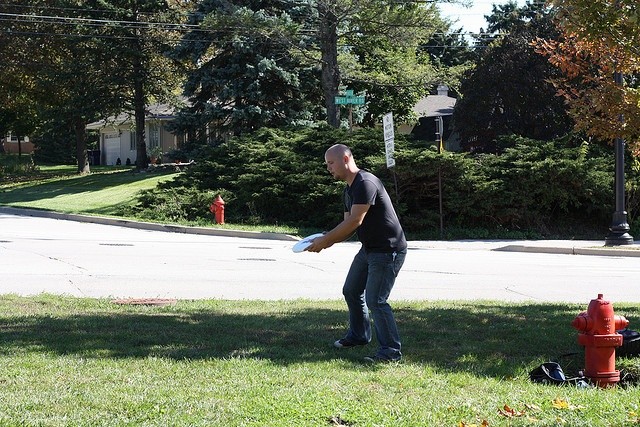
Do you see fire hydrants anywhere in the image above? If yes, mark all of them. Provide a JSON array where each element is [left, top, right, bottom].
[[210, 195, 225, 225], [571, 293, 630, 390]]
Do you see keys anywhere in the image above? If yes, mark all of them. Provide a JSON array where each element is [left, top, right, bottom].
[[393, 251, 398, 262]]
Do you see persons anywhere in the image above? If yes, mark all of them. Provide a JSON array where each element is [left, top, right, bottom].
[[303, 142, 407, 364]]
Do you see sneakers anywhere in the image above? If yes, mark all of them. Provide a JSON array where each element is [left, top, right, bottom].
[[334, 338, 356, 348], [364, 356, 389, 363]]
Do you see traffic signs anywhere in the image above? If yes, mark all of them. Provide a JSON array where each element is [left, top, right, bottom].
[[335, 90, 365, 105]]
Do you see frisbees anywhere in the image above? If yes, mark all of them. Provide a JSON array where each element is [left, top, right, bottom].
[[292, 234, 323, 253]]
